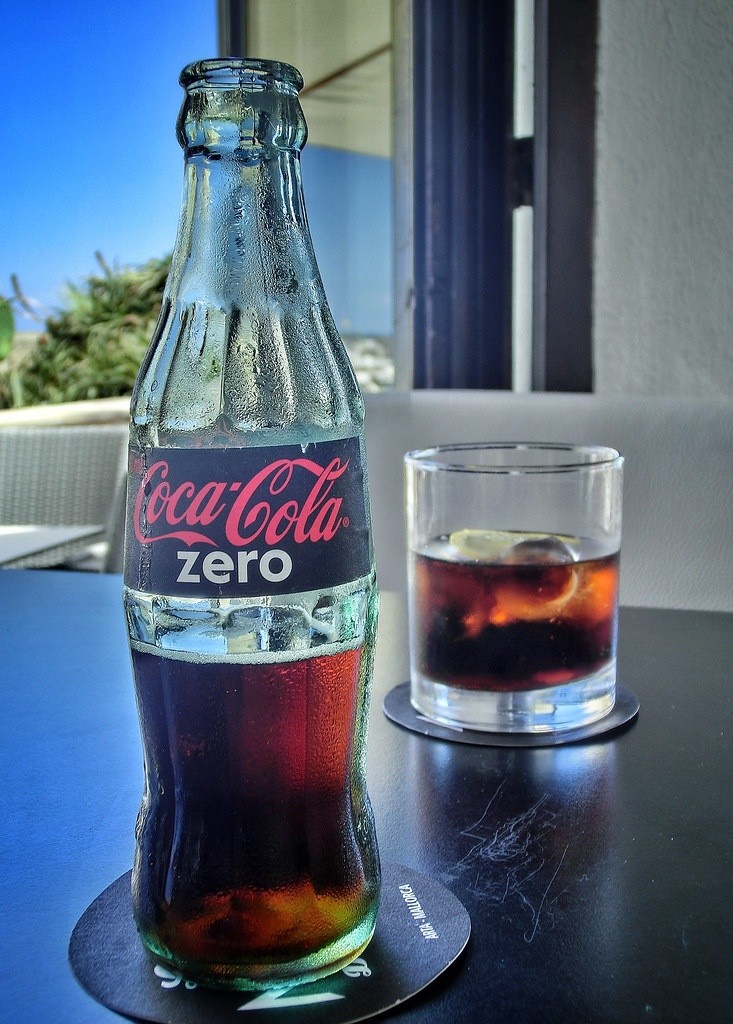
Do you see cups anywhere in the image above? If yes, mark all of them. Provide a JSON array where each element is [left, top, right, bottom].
[[404, 441, 625, 736]]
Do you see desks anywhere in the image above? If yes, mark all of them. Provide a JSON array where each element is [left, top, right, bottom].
[[0, 524, 106, 569], [0, 570, 733, 1024]]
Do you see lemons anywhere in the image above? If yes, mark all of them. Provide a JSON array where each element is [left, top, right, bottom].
[[450, 528, 579, 561]]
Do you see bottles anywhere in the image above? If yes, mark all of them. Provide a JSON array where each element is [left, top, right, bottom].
[[122, 57, 382, 987]]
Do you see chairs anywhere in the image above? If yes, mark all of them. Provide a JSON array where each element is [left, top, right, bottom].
[[0, 423, 127, 574]]
